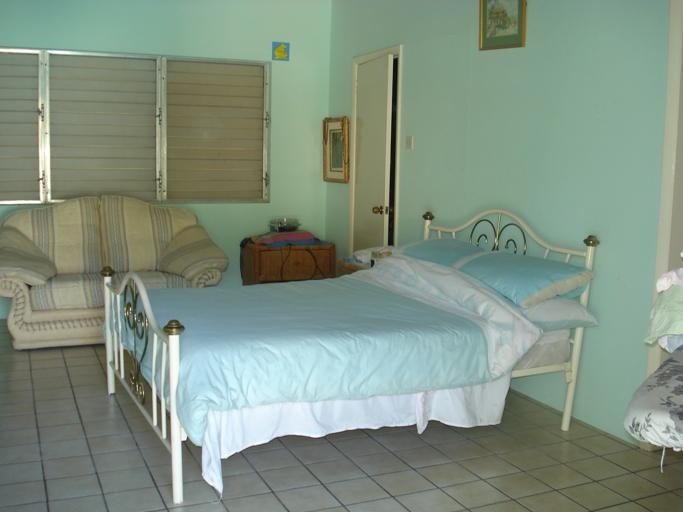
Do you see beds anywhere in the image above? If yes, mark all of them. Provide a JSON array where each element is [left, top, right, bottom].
[[99, 211, 602, 504]]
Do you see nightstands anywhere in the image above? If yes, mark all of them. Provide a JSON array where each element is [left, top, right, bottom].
[[338, 257, 371, 272]]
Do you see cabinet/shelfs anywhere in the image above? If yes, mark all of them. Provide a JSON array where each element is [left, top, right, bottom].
[[240, 238, 336, 284]]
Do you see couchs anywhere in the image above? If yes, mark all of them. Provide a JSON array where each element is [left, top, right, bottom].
[[1, 194, 229, 351]]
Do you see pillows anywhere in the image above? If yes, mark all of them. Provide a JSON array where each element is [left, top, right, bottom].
[[460, 253, 597, 308], [522, 299, 598, 329], [394, 241, 485, 271]]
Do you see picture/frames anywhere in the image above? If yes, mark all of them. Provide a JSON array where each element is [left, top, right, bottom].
[[479, 0, 526, 49], [323, 117, 349, 183]]
